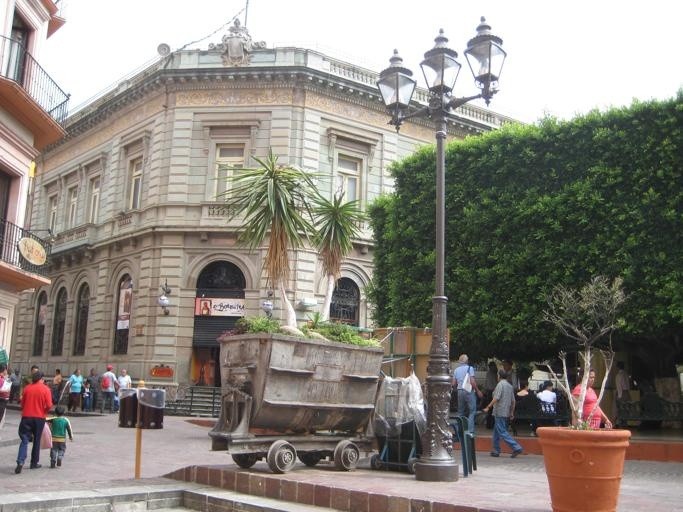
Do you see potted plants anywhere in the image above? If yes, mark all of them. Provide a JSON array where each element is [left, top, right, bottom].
[[533, 273, 631, 511]]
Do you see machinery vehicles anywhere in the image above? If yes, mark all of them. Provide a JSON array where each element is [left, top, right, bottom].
[[217, 315, 384, 476]]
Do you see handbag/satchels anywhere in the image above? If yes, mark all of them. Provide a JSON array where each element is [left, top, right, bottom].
[[32, 422, 54, 449], [0, 378, 12, 393], [127, 383, 131, 389], [462, 365, 473, 393]]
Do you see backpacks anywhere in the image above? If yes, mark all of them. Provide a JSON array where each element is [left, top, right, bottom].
[[102, 375, 110, 388]]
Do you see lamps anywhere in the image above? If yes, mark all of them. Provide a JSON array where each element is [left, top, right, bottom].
[[260, 288, 275, 319], [155, 275, 173, 316]]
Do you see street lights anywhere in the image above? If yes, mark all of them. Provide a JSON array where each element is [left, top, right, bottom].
[[377, 14, 507, 484]]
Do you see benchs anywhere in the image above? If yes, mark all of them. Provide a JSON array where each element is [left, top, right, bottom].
[[483, 392, 575, 436], [612, 394, 682, 429]]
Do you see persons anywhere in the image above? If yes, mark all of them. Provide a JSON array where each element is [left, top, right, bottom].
[[0, 362, 145, 428], [14, 370, 54, 474], [614, 361, 633, 429], [568, 367, 613, 428], [451, 354, 558, 458], [43, 406, 73, 468]]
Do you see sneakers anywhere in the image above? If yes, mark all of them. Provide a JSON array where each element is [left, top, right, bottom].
[[511, 449, 521, 457], [490, 452, 499, 457], [529, 431, 537, 436]]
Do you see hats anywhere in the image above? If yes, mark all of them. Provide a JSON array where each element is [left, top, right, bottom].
[[107, 365, 113, 369], [458, 354, 469, 363]]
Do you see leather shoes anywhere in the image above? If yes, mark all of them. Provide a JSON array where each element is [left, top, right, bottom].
[[15, 463, 22, 473], [30, 463, 42, 468]]
